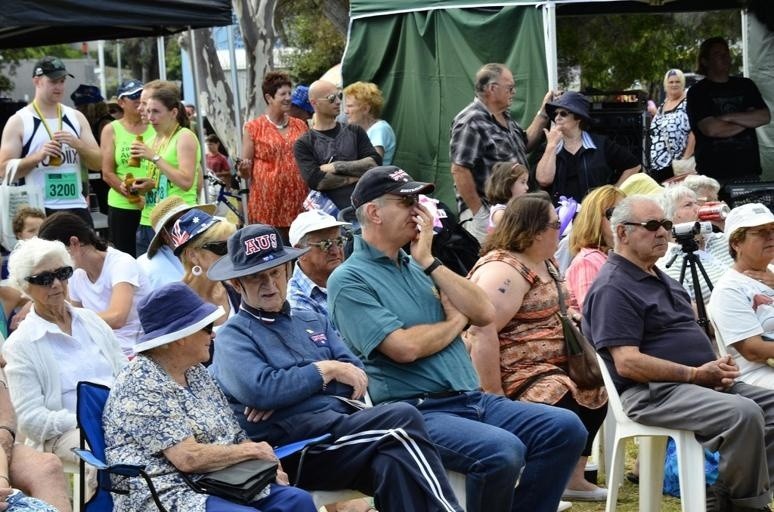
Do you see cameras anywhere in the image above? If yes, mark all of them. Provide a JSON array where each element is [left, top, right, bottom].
[[673, 220, 704, 253]]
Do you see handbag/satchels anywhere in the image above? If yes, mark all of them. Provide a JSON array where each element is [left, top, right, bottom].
[[196, 459, 278, 503], [545, 260, 605, 389], [672, 156, 696, 176], [303, 190, 340, 221], [0, 159, 46, 252]]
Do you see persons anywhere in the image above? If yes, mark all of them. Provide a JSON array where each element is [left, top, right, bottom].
[[0, 35, 774, 511]]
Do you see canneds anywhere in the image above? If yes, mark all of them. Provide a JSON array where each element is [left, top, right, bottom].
[[697, 202, 728, 222]]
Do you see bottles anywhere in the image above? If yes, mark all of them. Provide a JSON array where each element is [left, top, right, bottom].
[[47, 139, 64, 166], [128, 135, 144, 169], [125, 171, 142, 205]]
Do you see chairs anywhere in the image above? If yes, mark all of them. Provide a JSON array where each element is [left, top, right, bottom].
[[591, 351, 708, 512], [68, 379, 331, 511]]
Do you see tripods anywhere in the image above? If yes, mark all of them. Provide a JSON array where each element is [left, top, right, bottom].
[[675, 257, 713, 337]]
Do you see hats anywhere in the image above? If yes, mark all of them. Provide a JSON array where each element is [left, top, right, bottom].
[[117, 79, 143, 99], [289, 210, 353, 248], [545, 91, 593, 120], [350, 166, 436, 209], [207, 223, 309, 281], [33, 56, 74, 79], [147, 195, 216, 260], [170, 208, 222, 257], [292, 86, 315, 113], [131, 281, 225, 354], [724, 202, 774, 237]]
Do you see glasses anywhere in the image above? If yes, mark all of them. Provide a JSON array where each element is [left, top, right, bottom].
[[697, 198, 715, 201], [318, 90, 343, 103], [308, 238, 345, 250], [606, 207, 614, 220], [384, 194, 421, 206], [24, 267, 73, 285], [127, 92, 141, 99], [204, 322, 213, 332], [624, 220, 672, 231], [552, 111, 571, 116], [201, 242, 228, 256]]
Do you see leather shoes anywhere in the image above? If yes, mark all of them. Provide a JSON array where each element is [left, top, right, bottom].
[[557, 487, 607, 512]]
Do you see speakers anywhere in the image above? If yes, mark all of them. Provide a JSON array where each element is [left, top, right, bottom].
[[591, 112, 646, 181]]
